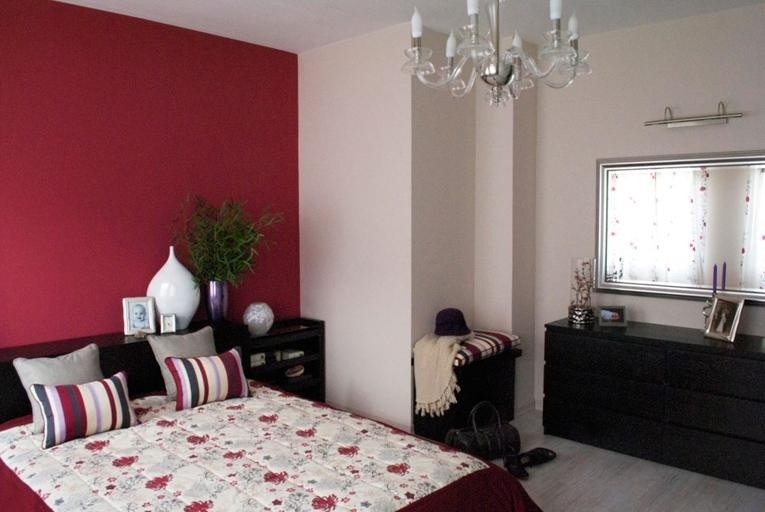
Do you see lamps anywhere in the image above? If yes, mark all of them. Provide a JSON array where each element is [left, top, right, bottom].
[[396, 0, 599, 108]]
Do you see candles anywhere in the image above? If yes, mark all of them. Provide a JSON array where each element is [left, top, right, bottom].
[[720, 262, 730, 291], [712, 262, 719, 293]]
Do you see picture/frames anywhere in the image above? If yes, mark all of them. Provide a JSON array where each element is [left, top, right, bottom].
[[703, 293, 746, 344], [595, 304, 628, 329], [121, 292, 157, 336]]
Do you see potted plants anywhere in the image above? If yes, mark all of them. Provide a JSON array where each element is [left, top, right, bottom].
[[569, 254, 597, 325], [176, 188, 289, 324]]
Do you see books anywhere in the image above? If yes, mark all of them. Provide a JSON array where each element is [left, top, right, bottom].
[[250, 349, 304, 368]]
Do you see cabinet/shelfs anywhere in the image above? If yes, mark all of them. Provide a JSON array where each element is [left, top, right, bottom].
[[540, 314, 765, 489], [410, 346, 525, 444]]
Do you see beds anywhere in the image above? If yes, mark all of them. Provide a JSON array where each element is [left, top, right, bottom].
[[0, 327, 550, 510]]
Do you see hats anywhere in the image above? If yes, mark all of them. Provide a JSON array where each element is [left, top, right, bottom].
[[434, 309, 470, 335]]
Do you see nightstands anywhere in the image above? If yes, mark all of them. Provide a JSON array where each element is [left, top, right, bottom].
[[239, 313, 327, 403]]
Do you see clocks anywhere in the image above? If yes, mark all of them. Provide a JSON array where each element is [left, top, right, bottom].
[[159, 311, 177, 334]]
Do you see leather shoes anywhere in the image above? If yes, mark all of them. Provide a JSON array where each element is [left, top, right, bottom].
[[520, 448, 555, 467], [503, 443, 528, 478]]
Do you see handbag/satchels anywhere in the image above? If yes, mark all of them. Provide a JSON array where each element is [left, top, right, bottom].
[[444, 401, 520, 461]]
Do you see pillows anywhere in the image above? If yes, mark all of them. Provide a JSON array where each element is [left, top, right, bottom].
[[160, 345, 256, 412], [9, 342, 103, 437], [26, 370, 143, 450], [144, 326, 218, 402], [445, 329, 522, 370]]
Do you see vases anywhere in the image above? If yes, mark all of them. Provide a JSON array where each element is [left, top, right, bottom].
[[144, 244, 199, 331], [242, 299, 277, 336]]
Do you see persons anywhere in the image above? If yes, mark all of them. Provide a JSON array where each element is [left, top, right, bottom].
[[713, 308, 730, 333], [131, 304, 149, 328], [602, 310, 620, 321]]
